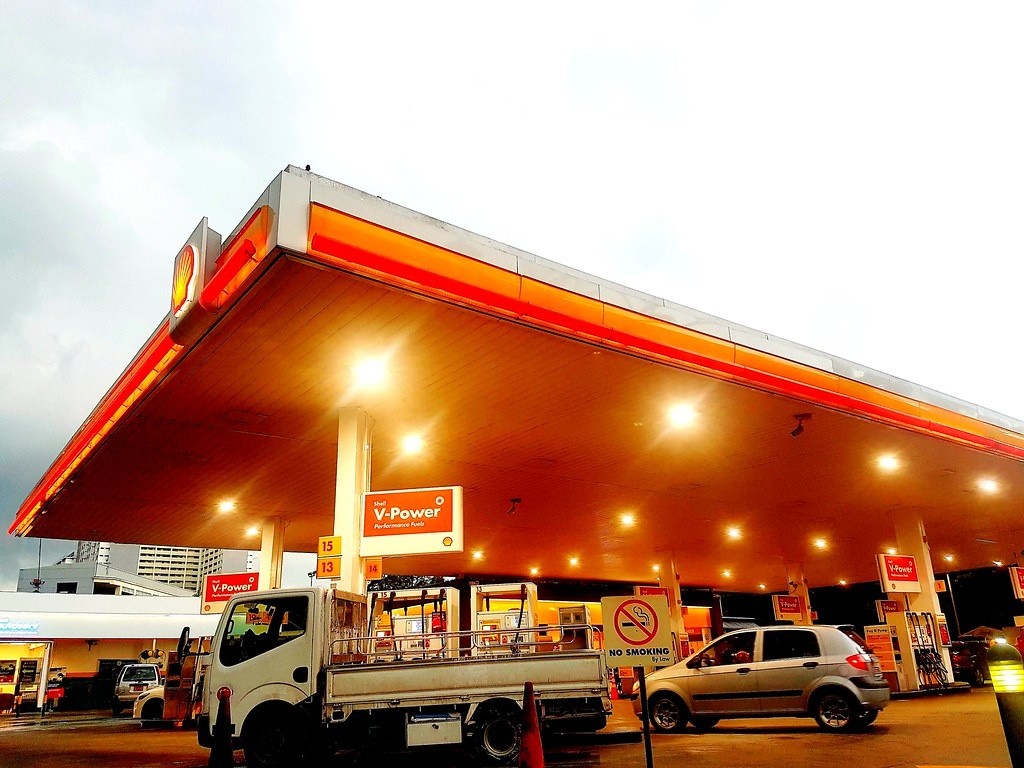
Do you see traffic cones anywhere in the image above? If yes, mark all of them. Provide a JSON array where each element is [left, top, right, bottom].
[[519, 681, 545, 768], [609, 676, 622, 700], [206, 689, 235, 768]]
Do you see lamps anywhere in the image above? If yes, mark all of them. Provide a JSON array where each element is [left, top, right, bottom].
[[987, 644, 1024, 768]]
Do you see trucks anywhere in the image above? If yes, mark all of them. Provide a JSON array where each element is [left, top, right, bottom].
[[176, 585, 613, 768]]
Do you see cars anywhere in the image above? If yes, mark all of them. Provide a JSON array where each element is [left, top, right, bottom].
[[631, 624, 891, 732], [949, 641, 989, 689], [133, 685, 165, 726], [112, 664, 166, 715]]
